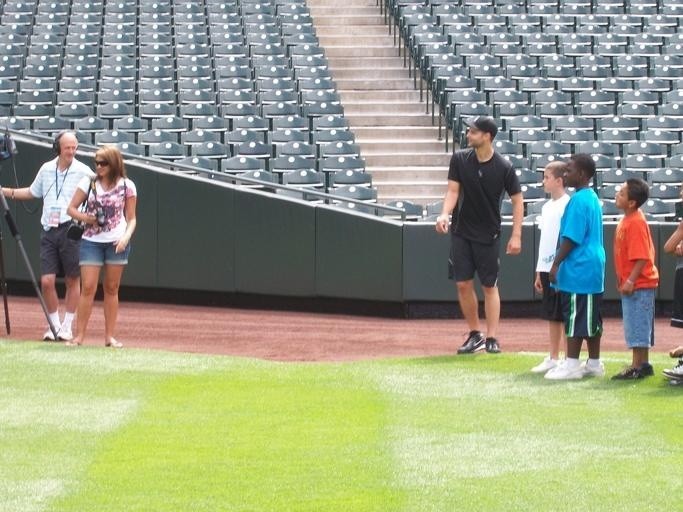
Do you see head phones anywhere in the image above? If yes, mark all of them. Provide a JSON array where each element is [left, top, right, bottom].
[[52, 130, 79, 155]]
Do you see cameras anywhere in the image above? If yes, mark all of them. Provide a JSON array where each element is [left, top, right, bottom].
[[1, 131, 19, 161], [95, 211, 108, 227]]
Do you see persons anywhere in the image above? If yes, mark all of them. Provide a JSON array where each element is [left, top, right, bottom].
[[611, 178, 660, 381], [530, 161, 575, 376], [66, 146, 137, 349], [435, 114, 525, 355], [543, 153, 607, 381], [664, 184, 683, 382], [0, 129, 97, 341]]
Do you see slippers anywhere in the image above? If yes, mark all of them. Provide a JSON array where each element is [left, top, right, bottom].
[[105, 337, 123, 348], [66, 341, 81, 346]]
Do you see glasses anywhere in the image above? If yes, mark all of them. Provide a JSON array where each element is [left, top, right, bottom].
[[94, 160, 109, 167]]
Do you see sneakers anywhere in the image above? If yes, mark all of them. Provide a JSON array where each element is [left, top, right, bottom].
[[580, 358, 605, 376], [640, 363, 654, 376], [457, 331, 486, 354], [56, 312, 75, 340], [43, 310, 61, 341], [532, 357, 563, 372], [544, 358, 584, 379], [611, 367, 644, 381], [486, 337, 501, 353], [662, 360, 683, 381]]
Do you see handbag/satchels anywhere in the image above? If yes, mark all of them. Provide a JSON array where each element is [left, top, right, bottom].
[[67, 178, 92, 240]]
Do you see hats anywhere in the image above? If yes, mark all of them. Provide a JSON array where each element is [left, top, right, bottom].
[[463, 116, 498, 137]]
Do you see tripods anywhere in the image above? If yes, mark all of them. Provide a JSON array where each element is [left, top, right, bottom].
[[2, 176, 60, 343]]
